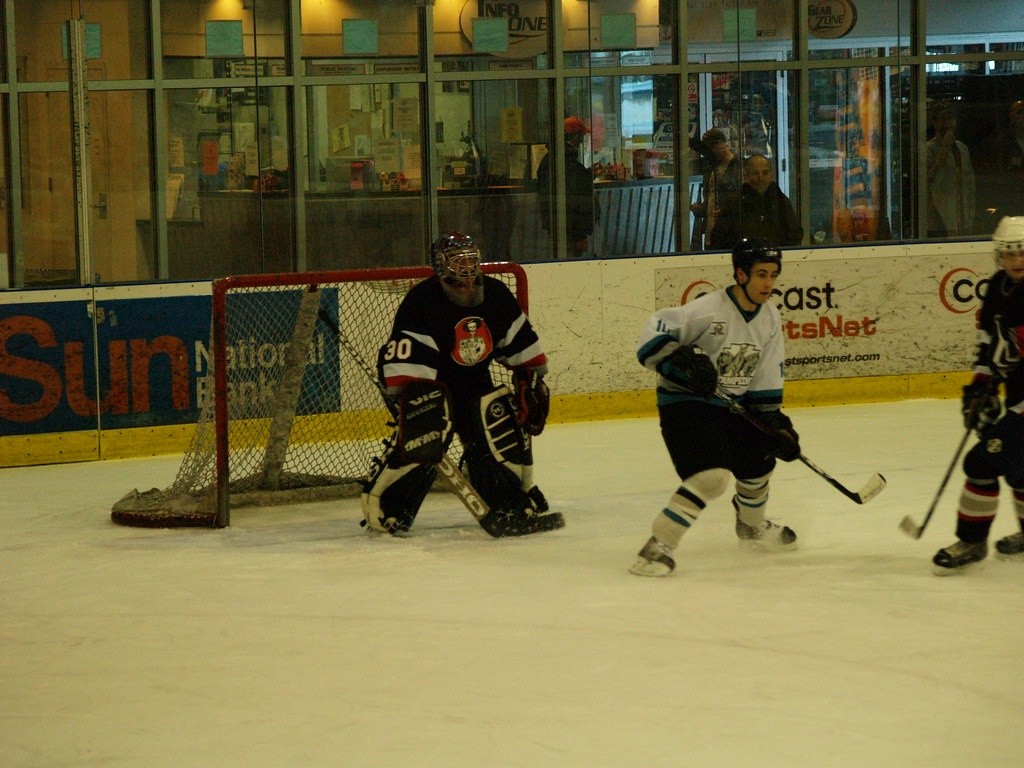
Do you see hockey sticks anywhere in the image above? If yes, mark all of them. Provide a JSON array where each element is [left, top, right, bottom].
[[710, 372, 887, 505], [897, 418, 974, 539], [322, 316, 566, 539]]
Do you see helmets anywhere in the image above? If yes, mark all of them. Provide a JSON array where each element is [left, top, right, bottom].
[[431, 231, 485, 308], [730, 231, 782, 277], [992, 215, 1024, 271]]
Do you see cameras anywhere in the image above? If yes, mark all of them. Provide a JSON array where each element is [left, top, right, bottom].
[[688, 137, 722, 167]]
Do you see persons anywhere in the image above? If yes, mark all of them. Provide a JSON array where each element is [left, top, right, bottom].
[[361, 229, 566, 537], [930, 214, 1024, 576], [475, 116, 517, 262], [926, 89, 1024, 238], [627, 232, 802, 578], [691, 128, 804, 248], [537, 116, 606, 259]]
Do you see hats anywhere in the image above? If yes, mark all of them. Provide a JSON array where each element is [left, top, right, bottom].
[[932, 101, 958, 120], [561, 116, 592, 135]]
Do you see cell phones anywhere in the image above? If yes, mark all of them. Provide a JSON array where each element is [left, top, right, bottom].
[[939, 126, 957, 150]]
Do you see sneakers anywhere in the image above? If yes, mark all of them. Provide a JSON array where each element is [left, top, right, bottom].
[[994, 531, 1024, 562], [628, 537, 676, 577], [932, 541, 989, 576], [735, 520, 799, 556]]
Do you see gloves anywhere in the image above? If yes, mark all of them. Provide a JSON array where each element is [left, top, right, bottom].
[[397, 382, 454, 465], [673, 343, 718, 397], [511, 373, 550, 436], [757, 412, 801, 463], [962, 373, 1003, 431]]
